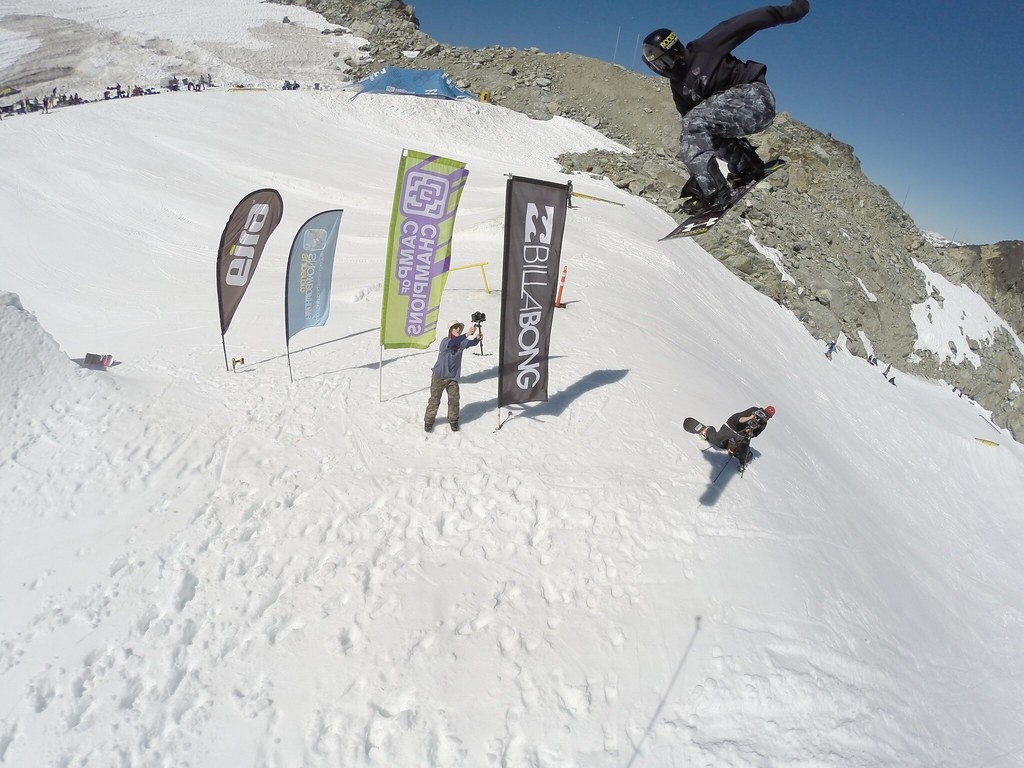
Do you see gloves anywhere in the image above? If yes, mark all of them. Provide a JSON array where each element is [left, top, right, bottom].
[[787, 0, 810, 22]]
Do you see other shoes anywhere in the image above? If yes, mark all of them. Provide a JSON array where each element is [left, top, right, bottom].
[[699, 426, 711, 440], [425, 423, 432, 432], [450, 422, 459, 431]]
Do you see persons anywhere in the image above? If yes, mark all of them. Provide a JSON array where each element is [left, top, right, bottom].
[[641, 1, 809, 213], [566, 179, 574, 208], [425, 319, 482, 433], [0, 74, 215, 121], [702, 405, 776, 464]]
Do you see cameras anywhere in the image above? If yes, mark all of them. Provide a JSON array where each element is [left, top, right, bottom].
[[749, 410, 765, 428], [472, 311, 486, 322]]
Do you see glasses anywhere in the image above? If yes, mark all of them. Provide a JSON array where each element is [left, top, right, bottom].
[[453, 327, 462, 329]]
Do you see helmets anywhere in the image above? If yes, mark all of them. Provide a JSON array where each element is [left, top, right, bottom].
[[642, 28, 685, 78]]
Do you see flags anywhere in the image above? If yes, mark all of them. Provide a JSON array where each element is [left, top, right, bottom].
[[380, 148, 470, 348], [286, 209, 343, 339], [498, 176, 569, 406], [216, 188, 284, 336]]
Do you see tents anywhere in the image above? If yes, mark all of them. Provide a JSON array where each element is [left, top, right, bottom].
[[336, 66, 478, 104]]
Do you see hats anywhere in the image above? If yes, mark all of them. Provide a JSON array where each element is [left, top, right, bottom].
[[765, 406, 775, 418], [448, 320, 465, 332]]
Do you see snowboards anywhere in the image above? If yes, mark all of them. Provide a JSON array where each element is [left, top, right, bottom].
[[656, 158, 789, 243]]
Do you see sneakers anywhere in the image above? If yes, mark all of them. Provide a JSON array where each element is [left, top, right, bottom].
[[682, 194, 732, 215], [731, 160, 764, 188]]
